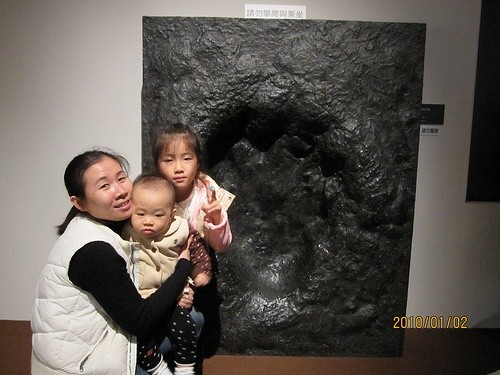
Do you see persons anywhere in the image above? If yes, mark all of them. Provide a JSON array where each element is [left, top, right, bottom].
[[29, 151, 195, 375], [119, 175, 213, 375], [151, 121, 236, 375]]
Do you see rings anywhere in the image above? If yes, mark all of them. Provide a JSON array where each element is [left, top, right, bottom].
[[186, 294, 189, 300]]
